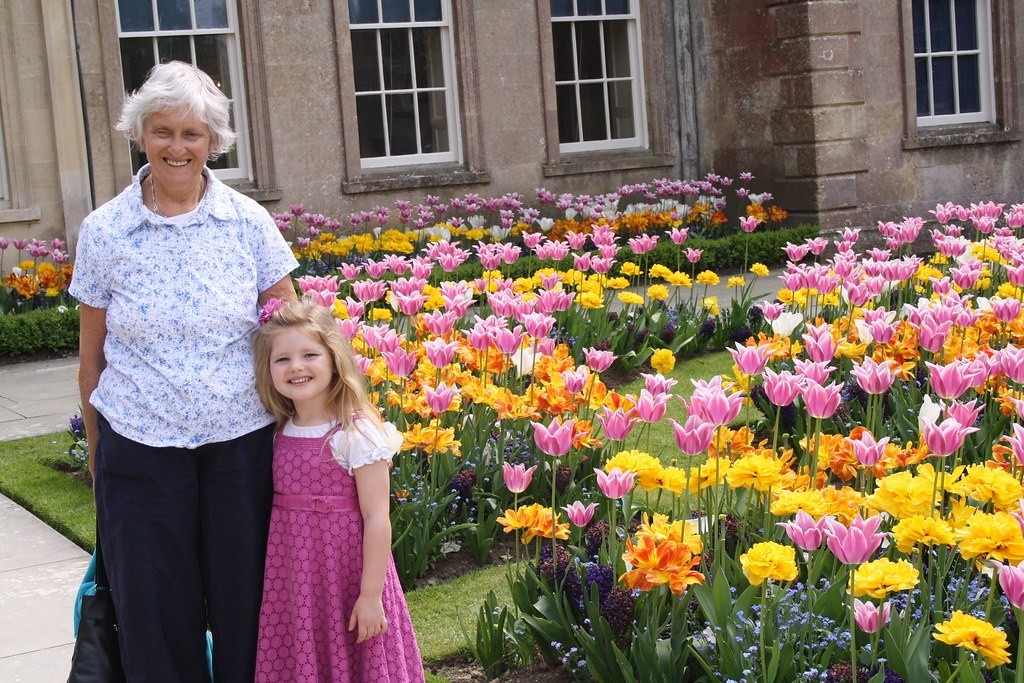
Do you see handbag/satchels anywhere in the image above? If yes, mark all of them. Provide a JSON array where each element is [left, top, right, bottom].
[[66, 515, 125, 683]]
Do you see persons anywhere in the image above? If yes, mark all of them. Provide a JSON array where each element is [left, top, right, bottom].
[[254, 301, 426, 683], [68, 61, 299, 683]]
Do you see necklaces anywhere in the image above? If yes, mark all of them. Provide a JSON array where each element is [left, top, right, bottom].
[[150, 176, 202, 217]]
